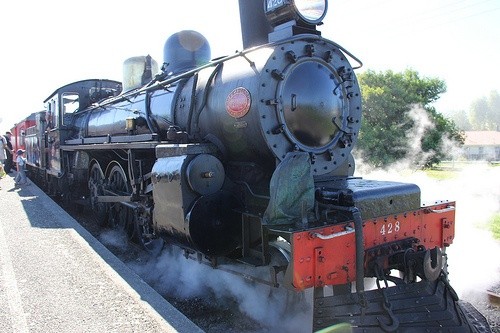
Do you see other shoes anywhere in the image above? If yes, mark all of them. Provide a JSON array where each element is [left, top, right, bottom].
[[15, 179, 31, 186]]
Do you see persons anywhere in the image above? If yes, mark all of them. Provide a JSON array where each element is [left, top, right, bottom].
[[15, 149, 32, 185], [46, 109, 52, 132], [0, 131, 15, 173]]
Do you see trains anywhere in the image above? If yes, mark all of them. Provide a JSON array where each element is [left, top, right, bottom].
[[0, 1, 489, 332]]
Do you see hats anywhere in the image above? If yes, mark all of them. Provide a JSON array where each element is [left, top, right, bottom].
[[16, 149, 25, 155], [5, 131, 12, 135]]
